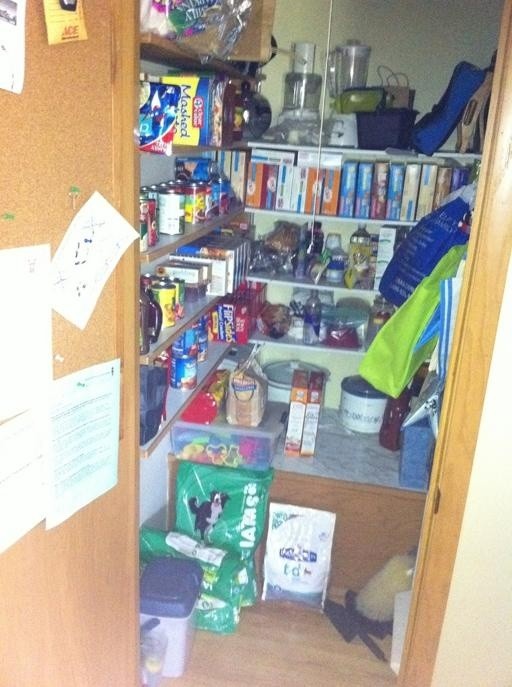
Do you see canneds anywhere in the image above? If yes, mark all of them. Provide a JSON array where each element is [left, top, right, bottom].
[[158, 191, 185, 235], [152, 286, 175, 328], [161, 277, 184, 319], [171, 324, 198, 357], [185, 184, 205, 223], [171, 356, 196, 390], [188, 312, 208, 361]]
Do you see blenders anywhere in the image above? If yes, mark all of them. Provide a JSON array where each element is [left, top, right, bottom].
[[322, 36, 372, 150]]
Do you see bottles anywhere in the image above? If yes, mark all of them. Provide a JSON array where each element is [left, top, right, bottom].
[[218, 167, 230, 215], [233, 90, 243, 141], [208, 161, 219, 216], [304, 222, 324, 278], [139, 202, 148, 253], [350, 223, 370, 261], [303, 289, 321, 346], [141, 179, 184, 199], [141, 198, 156, 246], [140, 289, 150, 355], [365, 296, 396, 353], [140, 276, 163, 342]]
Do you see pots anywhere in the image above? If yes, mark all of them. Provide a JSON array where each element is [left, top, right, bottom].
[[262, 359, 331, 404]]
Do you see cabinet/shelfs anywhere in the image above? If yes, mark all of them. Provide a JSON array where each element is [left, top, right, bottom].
[[0, 0, 512, 687]]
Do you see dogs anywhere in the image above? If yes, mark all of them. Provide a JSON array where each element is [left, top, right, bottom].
[[184, 491, 230, 548]]
